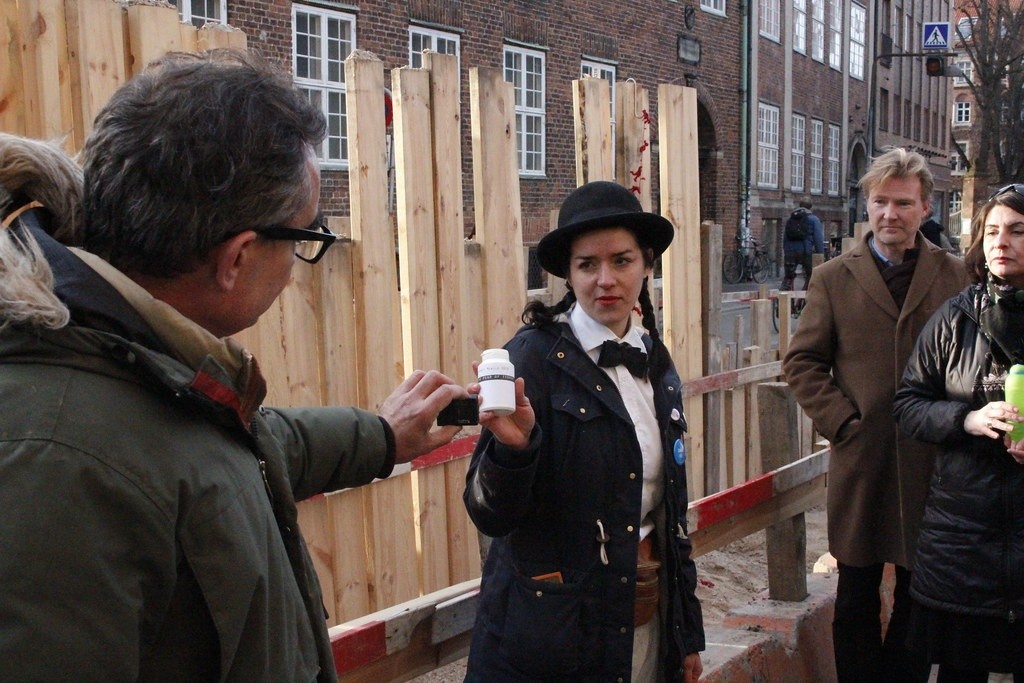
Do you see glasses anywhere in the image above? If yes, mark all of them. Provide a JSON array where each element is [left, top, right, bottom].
[[985, 183, 1024, 201], [213, 225, 337, 264]]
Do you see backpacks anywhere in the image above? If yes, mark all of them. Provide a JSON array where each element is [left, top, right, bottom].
[[785, 208, 813, 242]]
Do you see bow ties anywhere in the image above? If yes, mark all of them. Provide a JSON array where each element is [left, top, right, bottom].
[[598, 339, 648, 380]]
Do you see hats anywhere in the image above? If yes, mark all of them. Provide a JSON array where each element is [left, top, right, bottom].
[[537, 180, 676, 280]]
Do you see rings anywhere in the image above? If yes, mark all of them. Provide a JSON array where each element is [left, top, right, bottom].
[[988, 418, 993, 428]]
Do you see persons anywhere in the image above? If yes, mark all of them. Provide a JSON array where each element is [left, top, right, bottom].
[[782, 197, 824, 314], [782, 149, 970, 682], [919, 211, 945, 248], [0, 47, 471, 683], [465, 181, 706, 683], [893, 183, 1024, 683]]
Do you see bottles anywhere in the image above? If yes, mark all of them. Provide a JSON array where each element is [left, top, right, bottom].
[[477, 347, 516, 417], [1004, 363, 1024, 443]]
